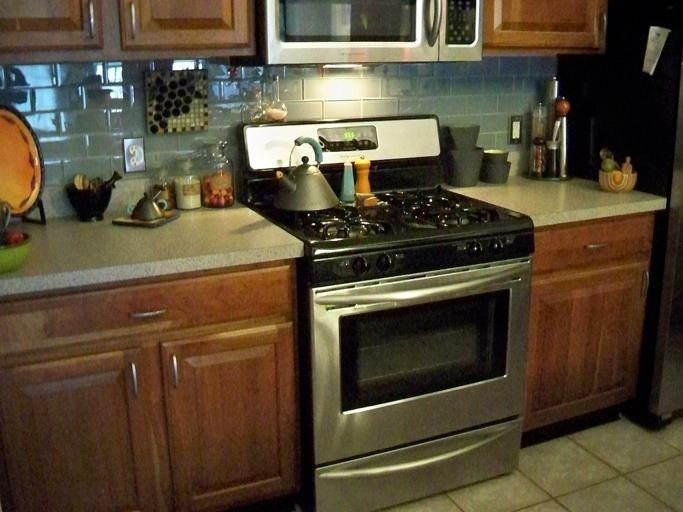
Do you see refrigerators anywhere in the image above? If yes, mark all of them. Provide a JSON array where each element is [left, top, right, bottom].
[[556, 0, 680, 428]]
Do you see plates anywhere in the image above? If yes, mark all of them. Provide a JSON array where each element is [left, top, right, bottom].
[[112, 211, 181, 228], [0, 104, 42, 219]]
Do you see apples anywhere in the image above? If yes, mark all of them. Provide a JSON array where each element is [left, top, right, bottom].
[[4, 231, 23, 246]]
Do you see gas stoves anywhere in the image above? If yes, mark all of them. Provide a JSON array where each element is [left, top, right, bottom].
[[248, 184, 538, 285]]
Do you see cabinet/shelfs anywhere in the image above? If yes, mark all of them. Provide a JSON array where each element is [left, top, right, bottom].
[[0, 0, 256, 65], [0, 257, 304, 512], [480, 0, 608, 56], [522, 209, 654, 437]]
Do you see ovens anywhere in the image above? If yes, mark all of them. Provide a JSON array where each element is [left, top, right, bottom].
[[300, 256, 534, 512]]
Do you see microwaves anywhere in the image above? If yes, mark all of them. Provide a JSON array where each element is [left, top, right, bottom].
[[228, 0, 484, 68]]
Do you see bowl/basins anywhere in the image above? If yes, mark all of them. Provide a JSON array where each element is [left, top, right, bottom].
[[62, 181, 111, 225], [446, 123, 511, 185], [0, 232, 30, 275], [597, 170, 638, 194]]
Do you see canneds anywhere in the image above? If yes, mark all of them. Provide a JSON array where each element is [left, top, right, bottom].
[[201, 140, 235, 206], [173, 152, 202, 210], [146, 153, 176, 210]]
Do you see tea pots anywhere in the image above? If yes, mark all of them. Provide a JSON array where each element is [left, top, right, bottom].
[[270, 135, 338, 216]]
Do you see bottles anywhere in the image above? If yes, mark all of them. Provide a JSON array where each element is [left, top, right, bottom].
[[528, 75, 570, 181], [340, 154, 373, 203], [152, 165, 174, 210], [250, 70, 289, 125], [172, 157, 201, 211], [196, 141, 233, 205]]
